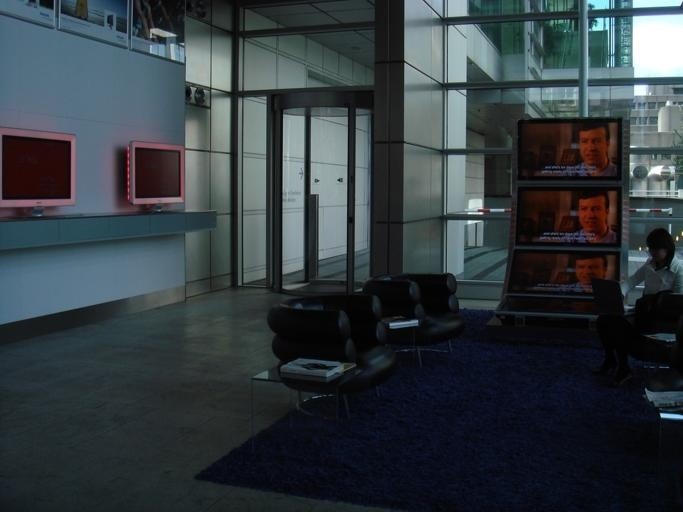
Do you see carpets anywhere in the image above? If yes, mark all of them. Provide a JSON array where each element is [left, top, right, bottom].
[[192, 307, 682, 512]]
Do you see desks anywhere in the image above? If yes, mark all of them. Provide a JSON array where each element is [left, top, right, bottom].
[[244, 363, 364, 456]]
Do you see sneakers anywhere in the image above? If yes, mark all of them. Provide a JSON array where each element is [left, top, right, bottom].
[[613, 367, 633, 385]]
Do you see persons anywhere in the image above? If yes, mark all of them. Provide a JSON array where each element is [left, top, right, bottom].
[[563, 121, 618, 176], [571, 255, 614, 293], [620, 228, 683, 300], [561, 191, 616, 244]]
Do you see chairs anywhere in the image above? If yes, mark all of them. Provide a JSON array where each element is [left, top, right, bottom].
[[265, 272, 465, 423]]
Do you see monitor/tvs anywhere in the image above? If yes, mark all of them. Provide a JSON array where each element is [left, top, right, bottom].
[[127, 140, 186, 212], [494, 116, 624, 329], [0, 126, 77, 218]]
